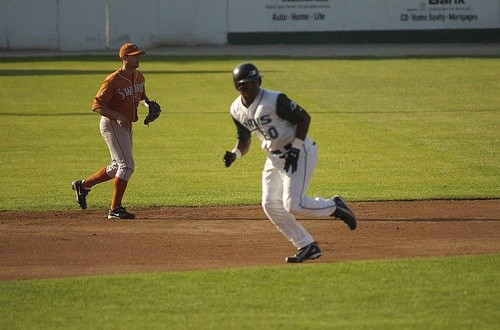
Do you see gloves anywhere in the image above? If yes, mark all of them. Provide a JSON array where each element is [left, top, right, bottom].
[[279, 147, 301, 173], [224, 151, 237, 167]]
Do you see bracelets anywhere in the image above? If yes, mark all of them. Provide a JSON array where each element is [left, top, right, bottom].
[[231, 148, 242, 160], [293, 138, 305, 150]]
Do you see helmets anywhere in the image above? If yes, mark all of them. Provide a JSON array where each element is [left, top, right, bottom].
[[232, 63, 263, 90]]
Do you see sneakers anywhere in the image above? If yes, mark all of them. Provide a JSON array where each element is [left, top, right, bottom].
[[108, 206, 135, 219], [71, 180, 91, 210], [330, 195, 357, 230], [286, 243, 322, 263]]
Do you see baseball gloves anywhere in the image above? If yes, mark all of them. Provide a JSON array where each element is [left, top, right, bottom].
[[143, 100, 161, 125]]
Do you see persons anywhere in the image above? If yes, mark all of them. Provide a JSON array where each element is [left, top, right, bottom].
[[224, 63, 358, 263], [72, 43, 162, 219]]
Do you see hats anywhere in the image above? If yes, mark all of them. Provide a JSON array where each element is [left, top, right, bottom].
[[119, 43, 145, 58]]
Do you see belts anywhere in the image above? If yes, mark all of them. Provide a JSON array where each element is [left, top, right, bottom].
[[273, 143, 292, 154]]
